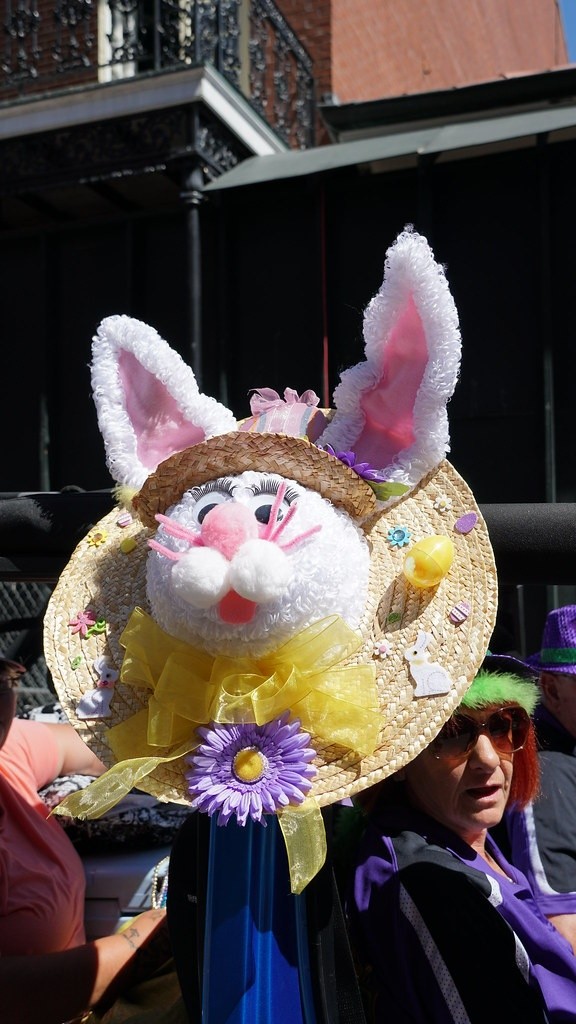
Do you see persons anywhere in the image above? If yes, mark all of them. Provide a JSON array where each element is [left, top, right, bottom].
[[0, 650, 168, 1024], [327, 674, 576, 1022], [504, 604, 576, 957]]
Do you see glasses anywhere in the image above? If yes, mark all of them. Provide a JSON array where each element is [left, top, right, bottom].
[[0, 673, 21, 692], [423, 708, 530, 757]]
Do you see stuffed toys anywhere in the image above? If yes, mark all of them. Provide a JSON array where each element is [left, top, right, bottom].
[[89, 222, 463, 658]]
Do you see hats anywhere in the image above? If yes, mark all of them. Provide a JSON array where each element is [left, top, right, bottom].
[[476, 655, 540, 684], [524, 604, 576, 676], [44, 231, 497, 895], [0, 649, 26, 672]]
